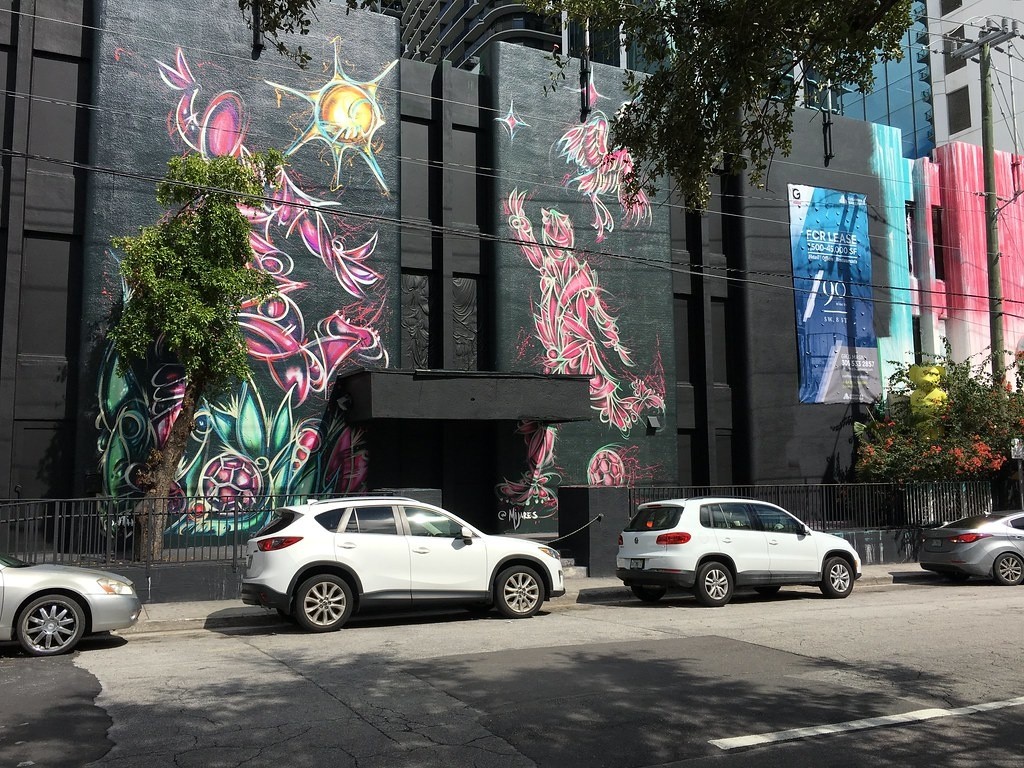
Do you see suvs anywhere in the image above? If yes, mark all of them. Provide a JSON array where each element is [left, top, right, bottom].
[[615, 496, 862, 608], [242, 495, 567, 634]]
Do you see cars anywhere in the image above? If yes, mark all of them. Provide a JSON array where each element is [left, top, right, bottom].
[[917, 510, 1024, 587], [0, 551, 143, 658]]
